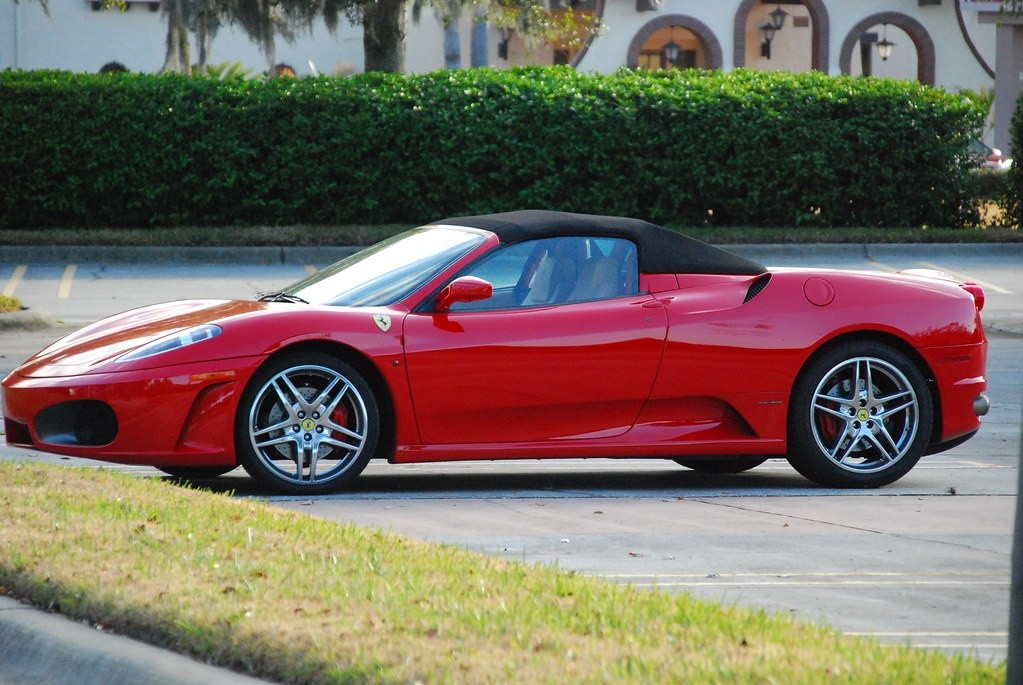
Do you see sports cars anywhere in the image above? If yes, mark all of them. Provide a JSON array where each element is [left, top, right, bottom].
[[1, 209, 991, 497]]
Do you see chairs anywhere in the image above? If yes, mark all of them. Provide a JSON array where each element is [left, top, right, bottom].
[[520, 257, 576, 306], [567, 256, 622, 301]]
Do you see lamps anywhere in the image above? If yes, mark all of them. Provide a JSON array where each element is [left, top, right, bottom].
[[660, 25, 683, 61], [759, 22, 776, 59], [874, 24, 895, 60], [768, 4, 789, 30]]
